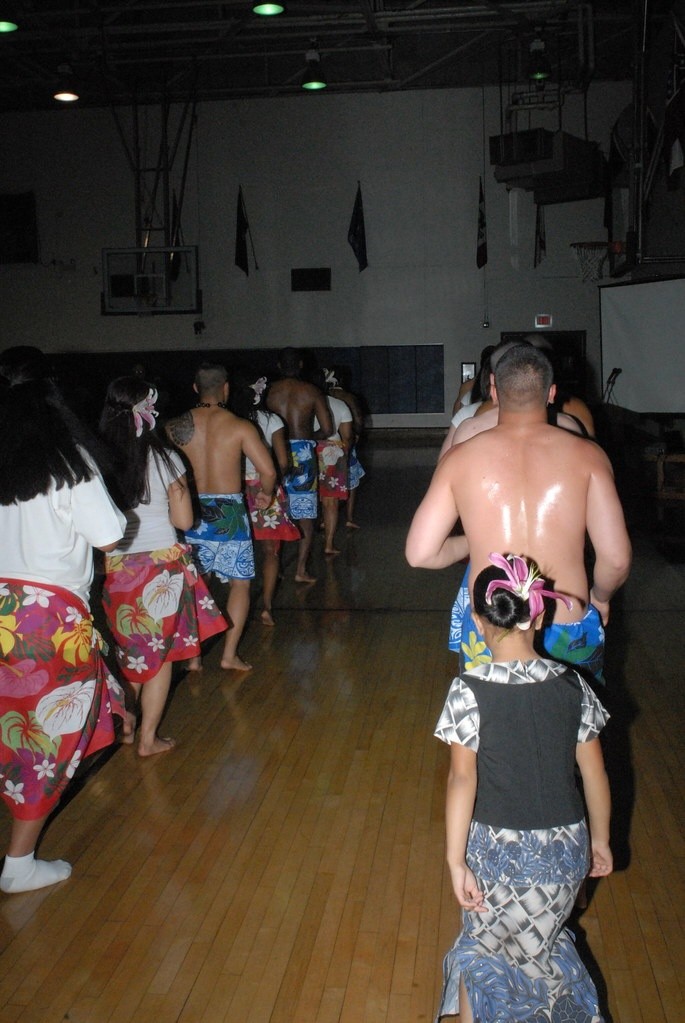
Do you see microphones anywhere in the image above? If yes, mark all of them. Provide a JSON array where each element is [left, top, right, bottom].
[[607, 368, 618, 384]]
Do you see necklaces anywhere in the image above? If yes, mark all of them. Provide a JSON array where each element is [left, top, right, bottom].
[[193, 402, 227, 409], [329, 387, 343, 390]]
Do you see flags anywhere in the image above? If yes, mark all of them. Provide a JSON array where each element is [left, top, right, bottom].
[[235, 192, 249, 277], [534, 205, 546, 267], [476, 181, 487, 269], [348, 188, 367, 272]]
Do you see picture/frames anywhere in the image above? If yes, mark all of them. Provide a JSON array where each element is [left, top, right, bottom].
[[101, 246, 198, 313]]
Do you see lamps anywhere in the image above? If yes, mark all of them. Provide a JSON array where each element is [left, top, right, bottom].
[[302, 58, 328, 90], [528, 49, 552, 79]]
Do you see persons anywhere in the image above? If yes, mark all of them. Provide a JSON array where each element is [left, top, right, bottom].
[[230, 376, 301, 626], [0, 345, 128, 893], [165, 365, 277, 671], [320, 366, 366, 529], [405, 348, 633, 695], [438, 334, 595, 463], [312, 368, 353, 554], [434, 552, 613, 1023], [265, 347, 333, 583], [95, 375, 230, 756]]
